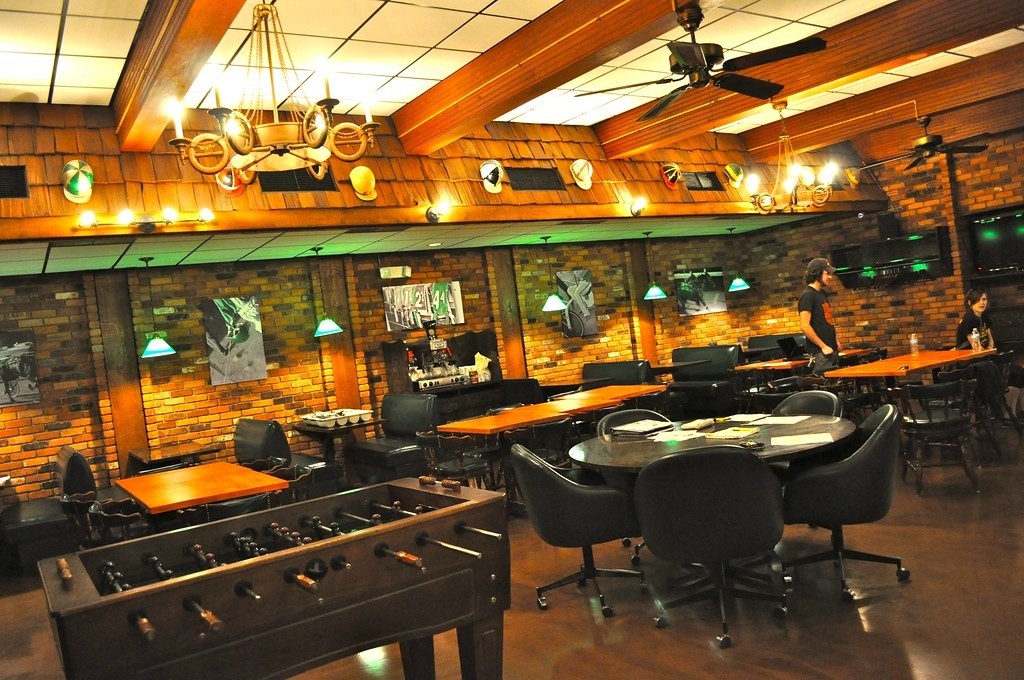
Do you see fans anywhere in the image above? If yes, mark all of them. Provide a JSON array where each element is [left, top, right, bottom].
[[858, 117, 992, 171], [574, 7, 826, 122]]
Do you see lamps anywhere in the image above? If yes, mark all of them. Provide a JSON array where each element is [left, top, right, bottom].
[[72, 0, 848, 361]]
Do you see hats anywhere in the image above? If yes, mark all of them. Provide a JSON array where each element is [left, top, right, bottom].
[[807, 258, 836, 275]]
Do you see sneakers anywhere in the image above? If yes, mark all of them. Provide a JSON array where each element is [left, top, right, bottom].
[[1001, 415, 1024, 429]]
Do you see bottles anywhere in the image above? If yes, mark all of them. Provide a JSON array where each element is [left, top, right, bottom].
[[971, 328, 981, 353], [909, 333, 918, 356]]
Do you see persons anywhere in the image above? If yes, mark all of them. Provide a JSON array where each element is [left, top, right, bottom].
[[798, 259, 840, 393], [956, 287, 1024, 386]]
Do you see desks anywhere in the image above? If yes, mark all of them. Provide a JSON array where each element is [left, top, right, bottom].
[[540, 376, 618, 393], [501, 400, 621, 434], [114, 460, 290, 514], [735, 348, 875, 385], [553, 385, 666, 413], [436, 412, 570, 519], [293, 418, 390, 460], [823, 349, 999, 403], [129, 441, 221, 468], [741, 346, 780, 355], [650, 359, 710, 373], [568, 414, 860, 592]]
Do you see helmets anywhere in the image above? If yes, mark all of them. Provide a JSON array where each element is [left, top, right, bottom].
[[844, 167, 862, 189], [215, 161, 245, 198], [800, 166, 815, 187], [479, 159, 504, 194], [570, 159, 593, 190], [723, 163, 744, 188], [660, 163, 682, 190], [61, 159, 94, 204], [349, 166, 377, 201]]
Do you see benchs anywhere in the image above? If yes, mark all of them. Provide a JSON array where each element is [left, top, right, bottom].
[[747, 334, 809, 362], [583, 360, 649, 391], [670, 344, 740, 400], [0, 375, 544, 564]]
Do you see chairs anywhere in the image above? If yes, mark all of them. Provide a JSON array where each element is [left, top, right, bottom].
[[58, 333, 1019, 648]]
